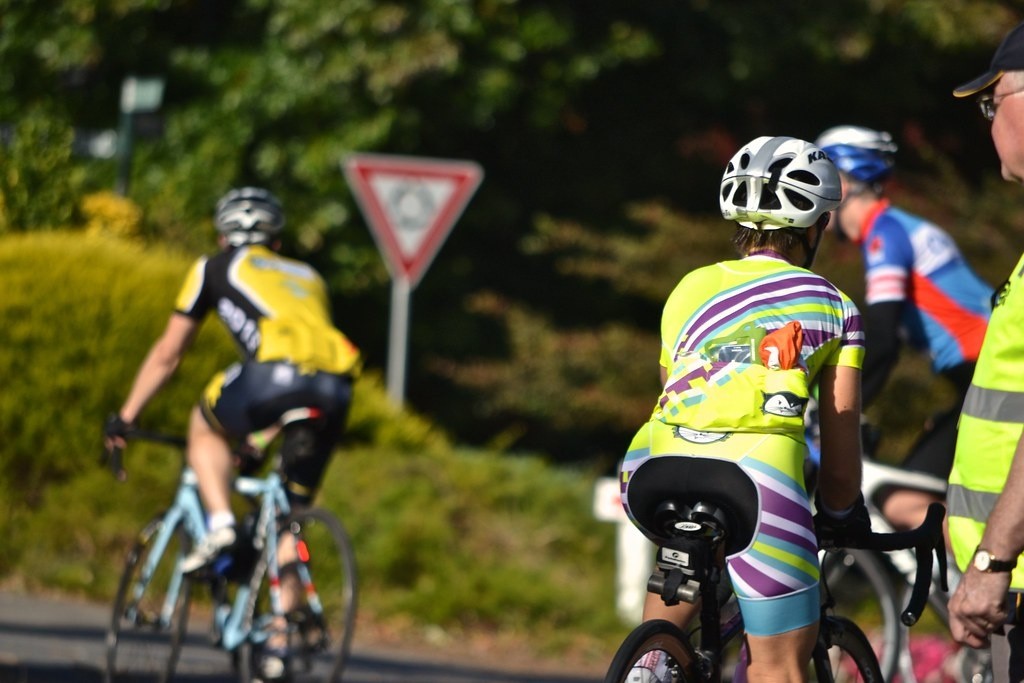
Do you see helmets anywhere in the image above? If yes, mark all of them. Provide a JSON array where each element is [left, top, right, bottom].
[[215, 188, 282, 235], [814, 126, 896, 179], [720, 136, 843, 231]]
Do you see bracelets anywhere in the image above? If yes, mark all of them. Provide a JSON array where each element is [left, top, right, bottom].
[[821, 490, 854, 516]]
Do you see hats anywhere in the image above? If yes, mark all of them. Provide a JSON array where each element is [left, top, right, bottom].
[[952, 22, 1024, 97]]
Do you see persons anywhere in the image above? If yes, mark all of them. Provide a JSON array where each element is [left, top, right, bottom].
[[619, 137, 871, 683], [815, 27, 1024, 683], [106, 187, 361, 683]]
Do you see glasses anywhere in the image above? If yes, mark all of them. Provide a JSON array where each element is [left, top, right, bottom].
[[978, 88, 1024, 120]]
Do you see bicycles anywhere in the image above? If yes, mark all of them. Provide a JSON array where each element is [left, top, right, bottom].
[[803, 434, 1012, 683], [599, 501, 902, 682], [95, 434, 361, 683]]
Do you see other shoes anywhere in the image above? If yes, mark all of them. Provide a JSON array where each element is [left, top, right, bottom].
[[181, 528, 239, 575]]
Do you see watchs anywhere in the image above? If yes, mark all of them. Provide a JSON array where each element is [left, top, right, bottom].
[[973, 545, 1018, 573]]
